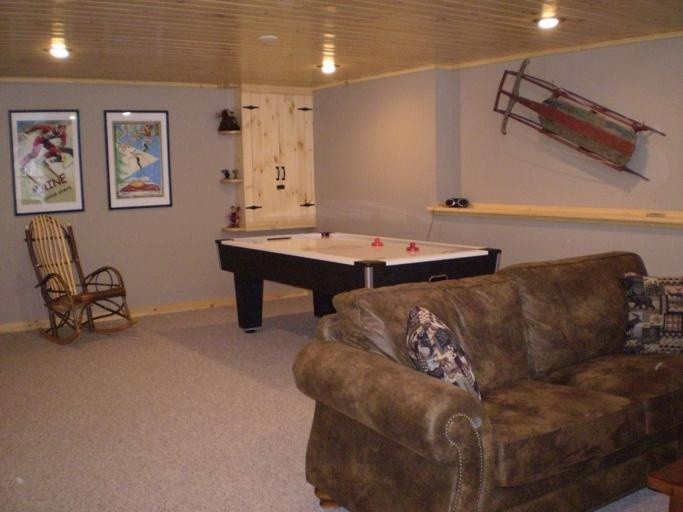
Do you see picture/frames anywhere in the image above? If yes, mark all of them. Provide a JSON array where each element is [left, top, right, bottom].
[[7, 109, 172, 216]]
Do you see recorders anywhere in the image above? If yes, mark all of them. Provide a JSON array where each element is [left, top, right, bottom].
[[447, 197, 469, 207]]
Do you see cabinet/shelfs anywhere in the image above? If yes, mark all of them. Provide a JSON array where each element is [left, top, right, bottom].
[[218, 92, 316, 233]]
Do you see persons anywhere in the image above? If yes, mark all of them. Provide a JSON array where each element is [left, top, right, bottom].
[[18, 121, 66, 177]]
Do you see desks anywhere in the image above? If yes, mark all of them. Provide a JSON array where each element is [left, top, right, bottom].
[[213, 233, 501, 332]]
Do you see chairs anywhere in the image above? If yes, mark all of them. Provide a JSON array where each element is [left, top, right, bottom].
[[24, 214, 139, 345]]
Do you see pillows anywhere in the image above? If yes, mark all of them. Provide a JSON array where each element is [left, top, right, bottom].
[[620, 271, 681, 356], [404, 304, 481, 400]]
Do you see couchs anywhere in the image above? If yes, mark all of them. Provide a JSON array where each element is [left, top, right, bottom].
[[292, 251, 681, 512]]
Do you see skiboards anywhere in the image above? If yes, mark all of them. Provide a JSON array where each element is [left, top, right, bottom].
[[18, 160, 61, 186]]
